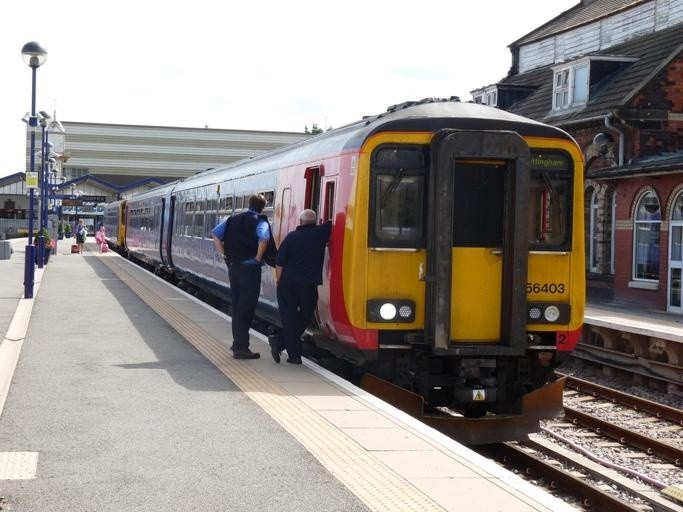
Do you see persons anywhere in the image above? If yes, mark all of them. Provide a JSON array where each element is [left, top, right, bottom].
[[95, 226, 106, 253], [268, 208, 334, 365], [210, 193, 277, 359], [75, 218, 88, 254]]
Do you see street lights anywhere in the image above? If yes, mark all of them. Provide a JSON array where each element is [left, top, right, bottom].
[[21, 39, 67, 298], [70, 183, 75, 238]]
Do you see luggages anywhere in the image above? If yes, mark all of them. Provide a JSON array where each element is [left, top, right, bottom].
[[71, 243, 78, 254], [102, 241, 109, 253]]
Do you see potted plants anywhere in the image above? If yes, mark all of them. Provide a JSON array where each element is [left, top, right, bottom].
[[35, 227, 52, 265], [58, 223, 64, 241], [65, 224, 71, 238]]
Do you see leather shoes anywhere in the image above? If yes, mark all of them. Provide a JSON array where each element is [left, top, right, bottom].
[[267, 333, 283, 363], [287, 356, 303, 364], [230, 346, 261, 360]]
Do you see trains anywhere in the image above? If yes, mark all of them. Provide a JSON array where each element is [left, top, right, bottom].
[[583, 198, 681, 283], [103, 97, 587, 443]]
